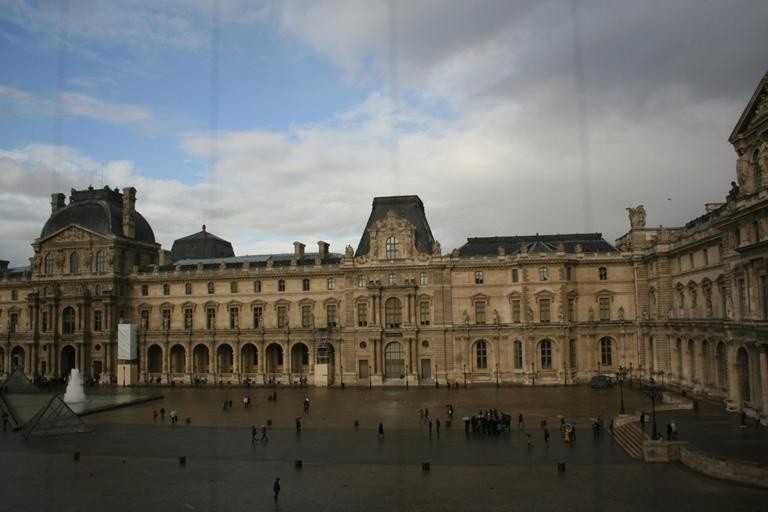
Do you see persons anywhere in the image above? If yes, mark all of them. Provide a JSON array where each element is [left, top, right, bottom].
[[1, 411, 9, 431], [303, 398, 311, 417], [169, 408, 177, 423], [251, 425, 258, 449], [223, 394, 252, 409], [639, 411, 645, 431], [644, 413, 650, 431], [755, 409, 760, 429], [377, 423, 385, 438], [272, 476, 282, 501], [72, 448, 76, 463], [296, 416, 304, 433], [260, 424, 268, 443], [151, 407, 157, 424], [160, 407, 165, 421], [667, 424, 673, 441], [671, 420, 678, 441], [418, 404, 616, 452], [740, 409, 746, 426], [76, 448, 80, 463]]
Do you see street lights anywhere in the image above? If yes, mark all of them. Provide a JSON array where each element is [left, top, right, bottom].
[[617, 362, 642, 414], [563, 361, 567, 385], [496, 363, 499, 388], [339, 364, 466, 388], [649, 377, 659, 439], [532, 362, 536, 387]]
